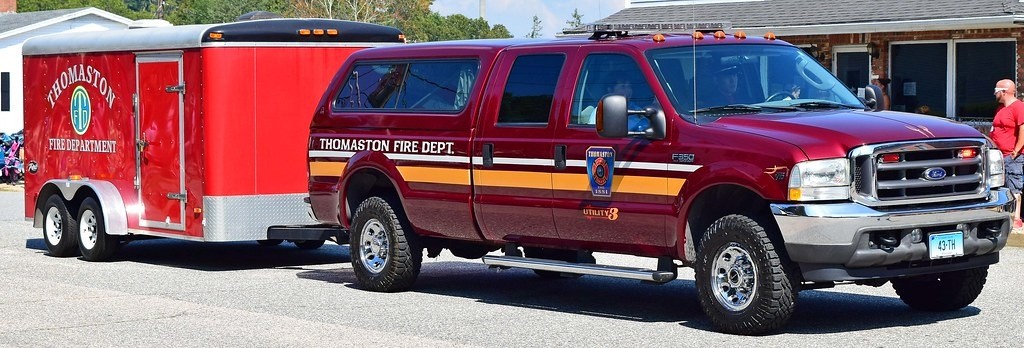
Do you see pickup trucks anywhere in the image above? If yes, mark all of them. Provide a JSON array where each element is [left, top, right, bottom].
[[304, 22, 1016, 337]]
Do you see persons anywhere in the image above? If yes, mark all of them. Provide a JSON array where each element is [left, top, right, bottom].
[[875, 79, 892, 109], [989, 79, 1024, 230], [709, 60, 801, 102], [589, 71, 657, 126]]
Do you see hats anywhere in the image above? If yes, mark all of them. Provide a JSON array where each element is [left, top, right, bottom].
[[873, 78, 891, 86], [712, 63, 740, 77]]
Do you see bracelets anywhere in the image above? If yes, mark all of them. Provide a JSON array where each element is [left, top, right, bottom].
[[1012, 152, 1016, 155]]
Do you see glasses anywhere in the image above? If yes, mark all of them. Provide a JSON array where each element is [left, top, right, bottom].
[[994, 87, 1008, 95]]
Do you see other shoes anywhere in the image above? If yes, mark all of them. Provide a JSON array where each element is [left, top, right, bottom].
[[1013, 218, 1023, 230]]
